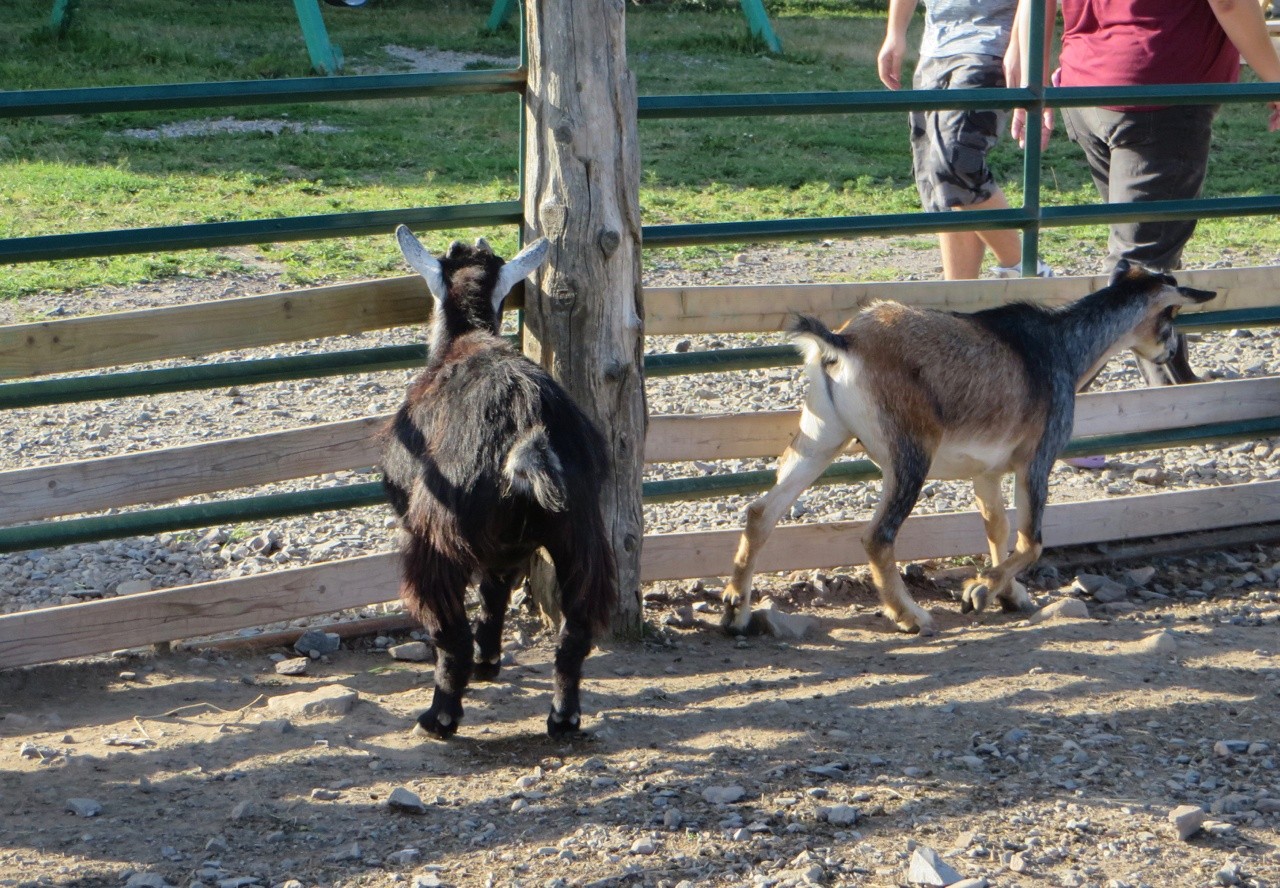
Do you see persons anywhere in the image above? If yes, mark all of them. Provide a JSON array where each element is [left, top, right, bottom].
[[874, 0, 1029, 282], [1006, 0, 1280, 393]]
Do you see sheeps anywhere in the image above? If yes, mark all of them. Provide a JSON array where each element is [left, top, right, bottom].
[[379, 222, 616, 740], [721, 258, 1216, 638]]
